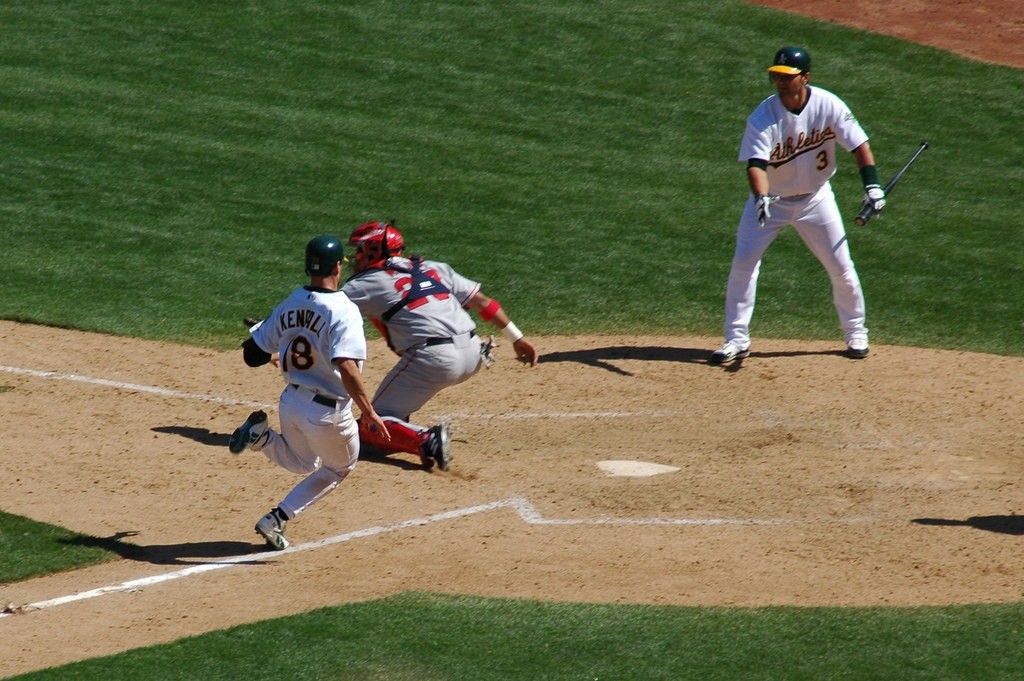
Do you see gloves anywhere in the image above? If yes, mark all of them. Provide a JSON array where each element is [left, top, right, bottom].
[[755, 196, 781, 228], [861, 185, 886, 216]]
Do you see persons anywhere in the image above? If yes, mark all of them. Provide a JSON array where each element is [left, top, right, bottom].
[[339, 221, 537, 472], [229, 234, 392, 550], [709, 48, 887, 366]]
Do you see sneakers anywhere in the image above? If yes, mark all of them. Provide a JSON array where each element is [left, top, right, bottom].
[[421, 425, 449, 470], [229, 410, 268, 455], [255, 510, 289, 551], [848, 339, 869, 358], [712, 341, 750, 363]]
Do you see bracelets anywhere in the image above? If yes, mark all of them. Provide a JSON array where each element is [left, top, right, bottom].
[[498, 320, 524, 344]]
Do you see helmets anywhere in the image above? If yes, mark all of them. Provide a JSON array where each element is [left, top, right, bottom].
[[305, 236, 348, 275], [766, 46, 811, 76], [348, 223, 404, 272]]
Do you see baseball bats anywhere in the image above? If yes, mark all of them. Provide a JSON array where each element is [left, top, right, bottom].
[[853, 141, 930, 228]]
